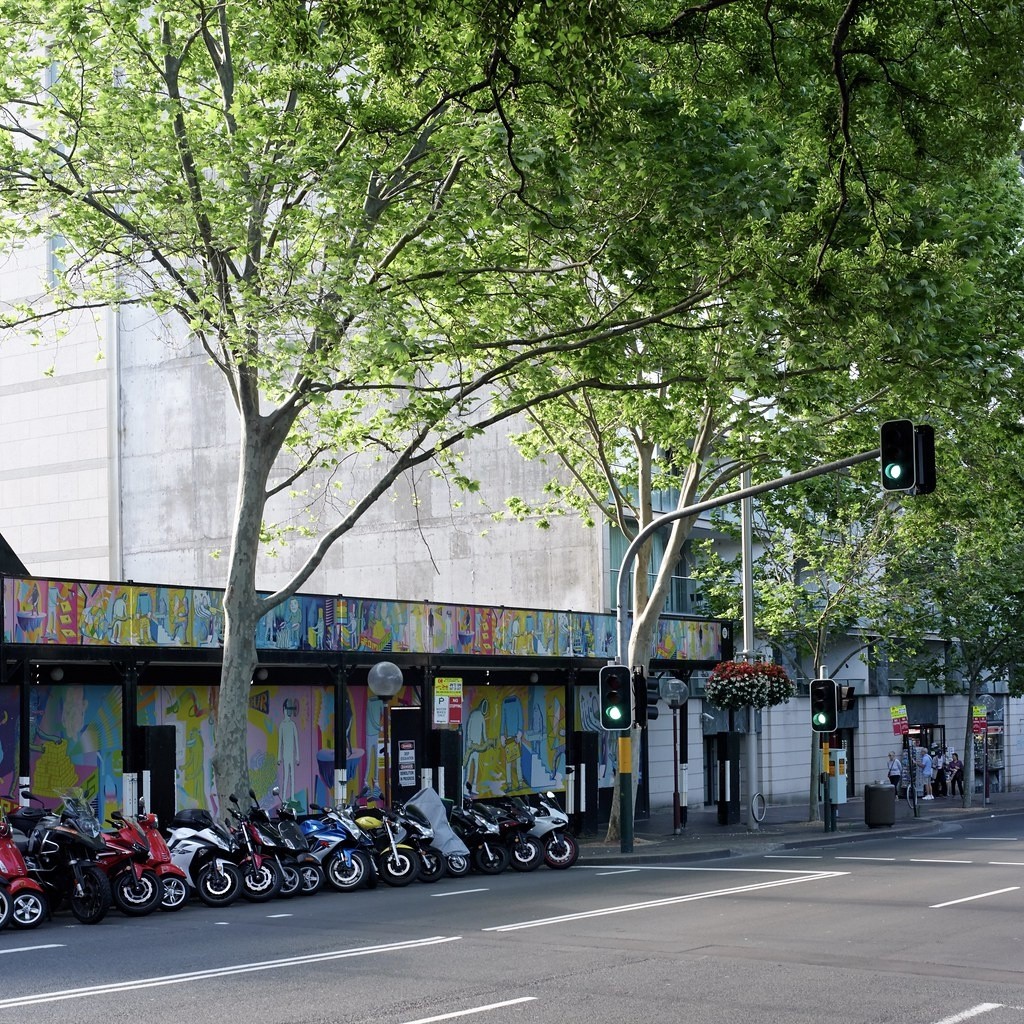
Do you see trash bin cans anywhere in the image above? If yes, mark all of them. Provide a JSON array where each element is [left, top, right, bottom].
[[865, 783, 895, 829]]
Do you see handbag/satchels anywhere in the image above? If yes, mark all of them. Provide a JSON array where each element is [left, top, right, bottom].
[[888, 769, 891, 777]]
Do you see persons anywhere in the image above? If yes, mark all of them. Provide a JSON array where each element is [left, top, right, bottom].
[[946, 753, 964, 799], [917, 748, 934, 800], [931, 750, 948, 799], [887, 751, 903, 802]]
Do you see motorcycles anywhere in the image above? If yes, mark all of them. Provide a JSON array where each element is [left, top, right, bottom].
[[0, 783, 578, 929]]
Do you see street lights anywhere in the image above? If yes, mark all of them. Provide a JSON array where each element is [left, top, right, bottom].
[[979, 694, 997, 804], [368, 663, 402, 818], [662, 680, 689, 835]]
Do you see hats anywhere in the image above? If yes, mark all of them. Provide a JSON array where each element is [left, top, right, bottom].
[[938, 749, 942, 754]]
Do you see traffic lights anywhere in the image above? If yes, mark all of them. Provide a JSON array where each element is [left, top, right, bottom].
[[809, 680, 838, 733], [599, 667, 633, 730], [838, 686, 856, 715], [635, 673, 660, 729], [881, 419, 917, 493]]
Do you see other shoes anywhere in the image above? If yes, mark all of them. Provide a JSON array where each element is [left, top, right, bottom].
[[952, 795, 956, 799], [944, 796, 947, 799], [922, 795, 934, 800], [961, 795, 964, 799], [895, 795, 898, 801]]
